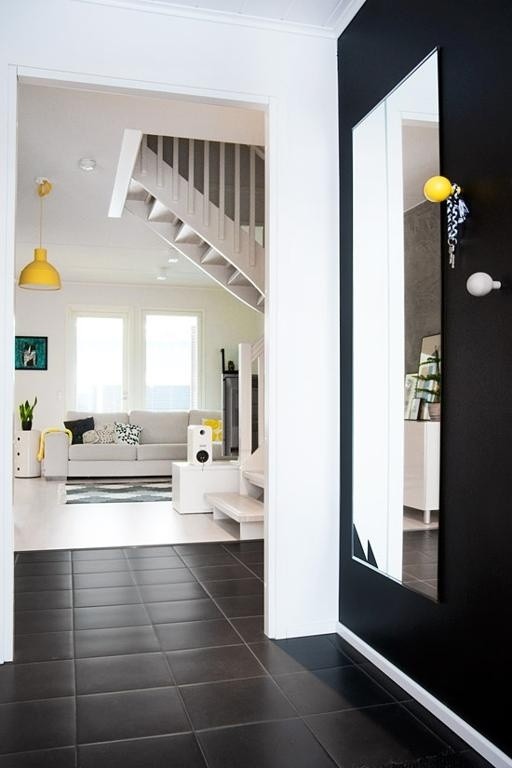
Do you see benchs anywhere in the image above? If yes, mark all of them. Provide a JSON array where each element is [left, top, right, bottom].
[[204, 491, 264, 540]]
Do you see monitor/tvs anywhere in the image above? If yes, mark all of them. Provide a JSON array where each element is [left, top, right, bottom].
[[221, 373, 258, 458]]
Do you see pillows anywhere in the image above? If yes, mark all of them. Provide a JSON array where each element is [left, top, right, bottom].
[[113, 420, 144, 446], [64, 416, 94, 444], [202, 418, 223, 442], [82, 423, 115, 444]]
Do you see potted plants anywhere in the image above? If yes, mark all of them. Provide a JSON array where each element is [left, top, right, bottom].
[[19, 396, 38, 430]]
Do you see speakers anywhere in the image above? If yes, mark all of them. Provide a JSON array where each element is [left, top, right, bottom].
[[187, 425, 212, 466]]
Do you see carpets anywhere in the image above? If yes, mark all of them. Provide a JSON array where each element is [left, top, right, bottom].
[[57, 481, 172, 504]]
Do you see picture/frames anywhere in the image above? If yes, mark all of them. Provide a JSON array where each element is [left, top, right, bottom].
[[15, 336, 48, 371]]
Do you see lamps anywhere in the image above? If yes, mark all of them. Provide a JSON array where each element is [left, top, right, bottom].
[[18, 179, 61, 291]]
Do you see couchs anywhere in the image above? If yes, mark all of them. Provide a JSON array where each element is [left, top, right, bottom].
[[44, 409, 224, 481]]
